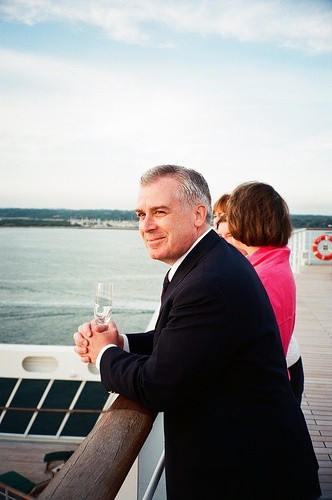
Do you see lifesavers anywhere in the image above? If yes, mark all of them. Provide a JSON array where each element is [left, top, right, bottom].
[[313, 234, 332, 260]]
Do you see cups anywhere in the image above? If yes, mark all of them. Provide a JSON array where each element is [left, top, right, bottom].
[[94, 283, 111, 332]]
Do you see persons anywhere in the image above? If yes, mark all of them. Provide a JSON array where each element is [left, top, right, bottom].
[[213, 182, 306, 407], [73, 165, 323, 500]]
